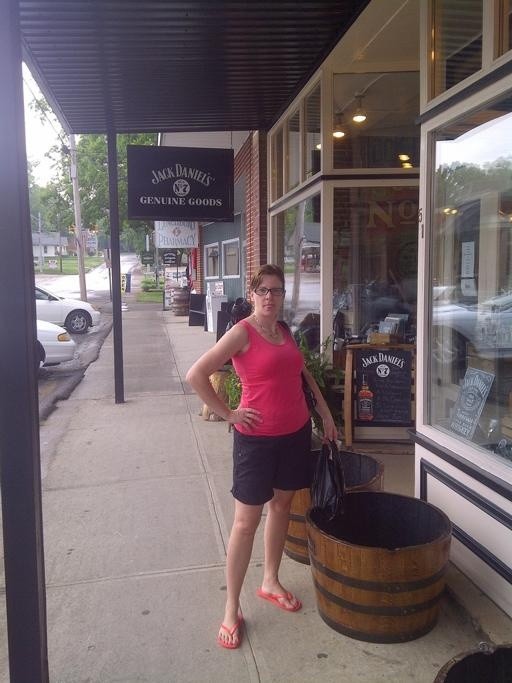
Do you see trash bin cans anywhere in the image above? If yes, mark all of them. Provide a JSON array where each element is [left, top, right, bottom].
[[124, 273, 131, 293], [189, 293, 206, 326]]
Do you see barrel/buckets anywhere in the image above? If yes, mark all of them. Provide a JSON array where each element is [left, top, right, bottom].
[[305, 489, 452, 645], [173, 289, 189, 315], [282, 446, 385, 567], [432, 639, 512, 683]]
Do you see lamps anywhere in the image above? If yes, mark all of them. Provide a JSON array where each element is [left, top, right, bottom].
[[351, 92, 367, 123], [332, 109, 346, 138]]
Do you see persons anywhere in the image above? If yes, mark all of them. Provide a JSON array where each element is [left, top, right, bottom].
[[184, 263, 339, 649]]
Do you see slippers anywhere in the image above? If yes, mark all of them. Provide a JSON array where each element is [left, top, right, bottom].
[[256, 587, 301, 611], [218, 615, 244, 649]]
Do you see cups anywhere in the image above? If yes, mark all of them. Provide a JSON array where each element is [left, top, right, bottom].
[[333, 338, 344, 351]]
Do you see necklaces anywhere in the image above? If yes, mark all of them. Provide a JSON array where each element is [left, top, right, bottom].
[[252, 314, 281, 338]]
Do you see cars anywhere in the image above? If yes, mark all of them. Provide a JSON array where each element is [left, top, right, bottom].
[[35, 284, 100, 371], [432, 286, 512, 368]]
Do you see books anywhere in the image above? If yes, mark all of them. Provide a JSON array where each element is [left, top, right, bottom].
[[378, 313, 410, 339]]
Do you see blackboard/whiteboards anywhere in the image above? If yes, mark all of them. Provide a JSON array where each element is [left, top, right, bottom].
[[355, 348, 412, 425]]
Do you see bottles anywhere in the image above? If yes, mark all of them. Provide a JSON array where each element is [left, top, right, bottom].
[[357, 373, 374, 421], [488, 419, 501, 452]]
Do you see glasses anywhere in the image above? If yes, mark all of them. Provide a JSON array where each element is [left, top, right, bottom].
[[252, 287, 286, 296]]
